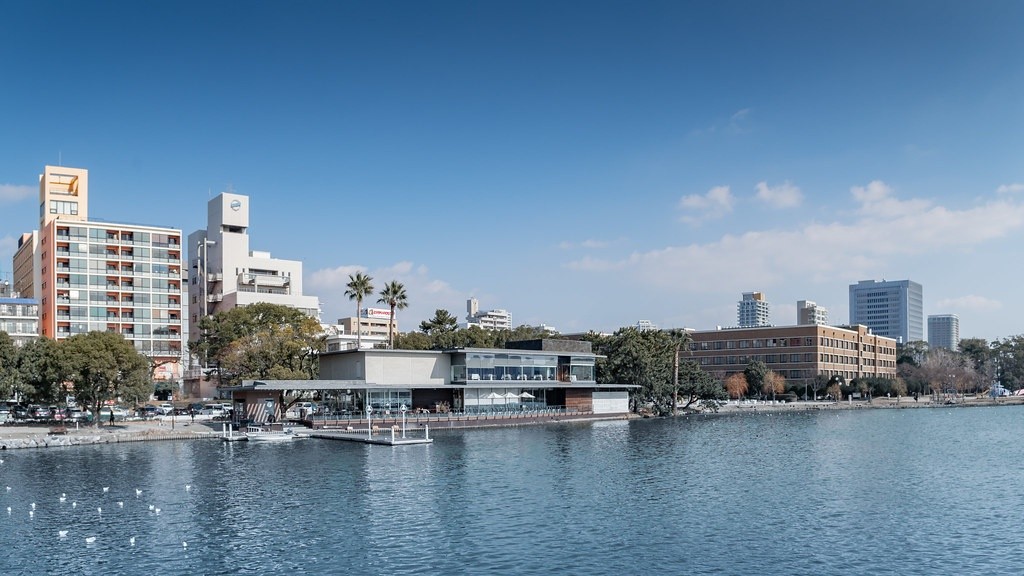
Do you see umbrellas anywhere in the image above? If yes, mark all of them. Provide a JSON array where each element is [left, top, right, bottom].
[[484, 392, 502, 406], [503, 392, 517, 407], [519, 392, 535, 404]]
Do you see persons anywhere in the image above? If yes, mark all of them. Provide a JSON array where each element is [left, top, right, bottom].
[[109, 410, 115, 426], [189, 408, 195, 421]]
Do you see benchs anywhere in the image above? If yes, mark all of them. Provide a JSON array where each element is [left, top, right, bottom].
[[48, 426, 69, 435]]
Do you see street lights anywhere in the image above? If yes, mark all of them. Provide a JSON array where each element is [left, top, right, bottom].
[[130, 394, 138, 410], [805, 379, 808, 401]]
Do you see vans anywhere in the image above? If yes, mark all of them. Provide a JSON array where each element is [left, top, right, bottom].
[[198, 404, 223, 415], [293, 402, 312, 412]]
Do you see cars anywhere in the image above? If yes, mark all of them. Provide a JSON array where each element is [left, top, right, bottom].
[[134, 406, 159, 416], [222, 403, 233, 410], [173, 405, 188, 415], [311, 403, 317, 411], [10, 401, 87, 420], [157, 403, 174, 415], [186, 403, 204, 414]]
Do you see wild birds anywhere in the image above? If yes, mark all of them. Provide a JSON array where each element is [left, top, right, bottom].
[[684, 411, 841, 426], [0, 458, 193, 548], [0, 433, 56, 440]]
